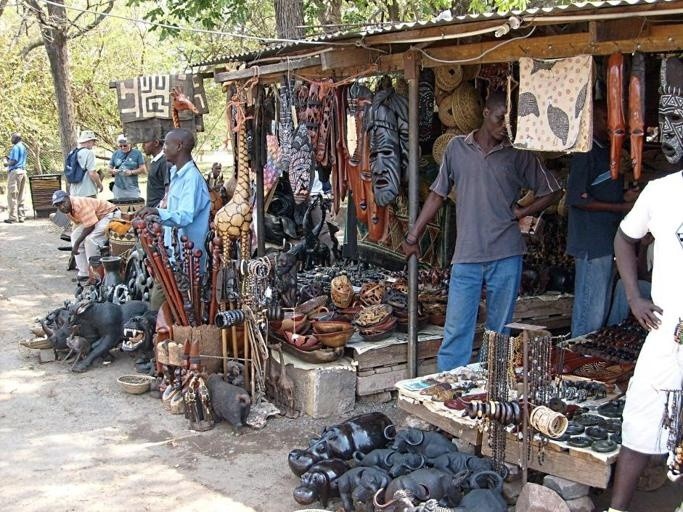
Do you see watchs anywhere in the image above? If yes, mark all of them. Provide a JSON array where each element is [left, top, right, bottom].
[[405, 238, 418, 246]]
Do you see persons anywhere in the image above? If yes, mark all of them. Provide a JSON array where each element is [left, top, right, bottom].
[[52, 191, 122, 285], [4, 133, 27, 223], [203, 162, 225, 192], [405, 89, 563, 373], [603, 169, 682, 510], [142, 141, 172, 208], [136, 126, 211, 311], [69, 131, 103, 198], [108, 134, 146, 198], [564, 98, 641, 337]]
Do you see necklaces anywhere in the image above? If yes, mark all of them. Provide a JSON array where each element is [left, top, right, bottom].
[[481, 328, 567, 472], [241, 276, 269, 307]]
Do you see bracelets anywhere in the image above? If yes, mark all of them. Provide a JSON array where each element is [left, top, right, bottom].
[[408, 232, 418, 239], [467, 401, 524, 423], [267, 306, 283, 321], [240, 255, 277, 279], [215, 310, 244, 329], [550, 416, 622, 453], [530, 406, 567, 438]]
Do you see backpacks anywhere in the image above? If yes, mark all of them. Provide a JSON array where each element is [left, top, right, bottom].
[[64, 147, 90, 184]]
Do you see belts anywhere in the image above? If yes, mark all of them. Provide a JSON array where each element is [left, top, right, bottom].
[[112, 207, 120, 212]]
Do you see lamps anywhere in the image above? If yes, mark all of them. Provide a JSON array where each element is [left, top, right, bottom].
[[495, 17, 520, 37]]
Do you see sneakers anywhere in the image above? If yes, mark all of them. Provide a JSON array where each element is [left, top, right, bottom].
[[52, 190, 67, 206]]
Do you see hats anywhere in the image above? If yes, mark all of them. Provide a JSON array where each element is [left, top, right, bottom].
[[119, 144, 128, 148], [75, 129, 99, 143]]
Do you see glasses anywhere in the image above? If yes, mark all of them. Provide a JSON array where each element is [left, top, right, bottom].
[[4, 219, 17, 224]]
